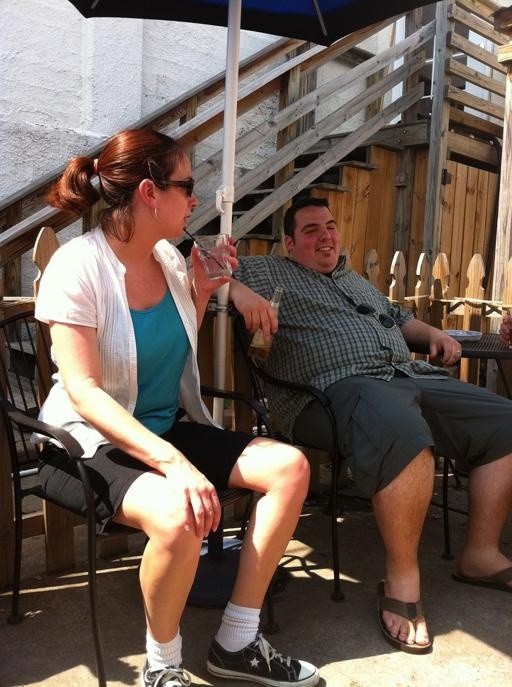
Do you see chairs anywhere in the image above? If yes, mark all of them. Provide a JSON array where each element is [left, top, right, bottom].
[[1, 308, 276, 687], [229, 308, 454, 601]]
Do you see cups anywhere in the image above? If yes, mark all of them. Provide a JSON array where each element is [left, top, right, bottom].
[[192, 233, 232, 280]]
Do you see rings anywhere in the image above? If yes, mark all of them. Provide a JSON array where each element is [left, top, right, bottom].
[[456, 351, 461, 353]]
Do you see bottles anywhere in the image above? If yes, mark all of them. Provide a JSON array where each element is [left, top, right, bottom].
[[246, 285, 284, 362]]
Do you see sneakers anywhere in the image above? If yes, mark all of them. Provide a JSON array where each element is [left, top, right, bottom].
[[206, 631, 320, 687], [141, 658, 192, 687]]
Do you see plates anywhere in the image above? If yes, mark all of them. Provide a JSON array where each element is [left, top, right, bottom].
[[441, 329, 483, 341]]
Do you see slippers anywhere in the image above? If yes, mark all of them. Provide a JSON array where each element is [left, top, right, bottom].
[[451, 566, 512, 593], [376, 578, 433, 655]]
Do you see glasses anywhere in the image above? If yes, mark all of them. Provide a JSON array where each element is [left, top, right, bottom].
[[157, 177, 195, 199], [355, 303, 395, 329]]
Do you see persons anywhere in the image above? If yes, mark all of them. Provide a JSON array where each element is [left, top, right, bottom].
[[27, 127, 322, 687], [499, 314, 512, 350], [208, 196, 512, 652]]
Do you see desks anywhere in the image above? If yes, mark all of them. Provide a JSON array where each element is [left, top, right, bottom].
[[403, 331, 511, 400]]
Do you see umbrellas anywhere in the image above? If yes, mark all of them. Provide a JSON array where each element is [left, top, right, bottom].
[[68, 0, 442, 556]]
